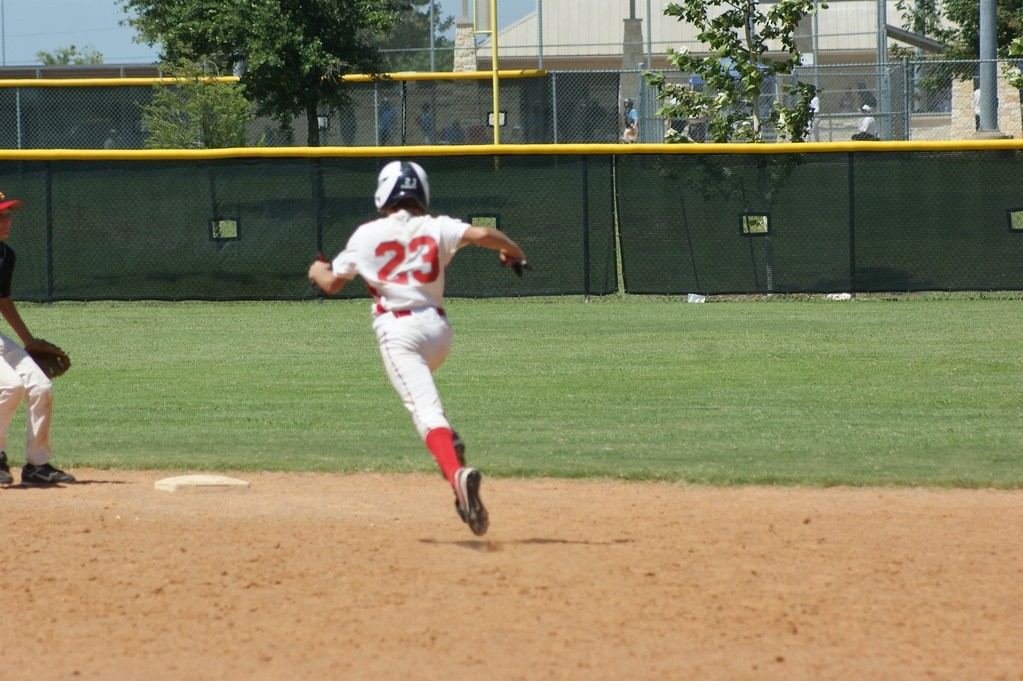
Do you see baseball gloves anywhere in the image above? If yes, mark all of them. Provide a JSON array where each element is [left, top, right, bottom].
[[26, 337, 71, 377]]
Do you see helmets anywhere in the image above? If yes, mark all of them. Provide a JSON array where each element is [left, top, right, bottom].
[[373, 159, 430, 214], [624, 97, 634, 108]]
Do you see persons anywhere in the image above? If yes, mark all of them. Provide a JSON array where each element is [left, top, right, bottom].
[[104, 130, 122, 147], [839, 79, 887, 142], [0, 193, 77, 491], [377, 96, 646, 145], [341, 93, 357, 147], [666, 82, 822, 143], [974, 86, 998, 130], [308, 160, 531, 537]]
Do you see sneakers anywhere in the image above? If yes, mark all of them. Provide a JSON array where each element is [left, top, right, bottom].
[[20, 463, 76, 487], [452, 466, 490, 537], [441, 430, 466, 482], [0, 451, 14, 484]]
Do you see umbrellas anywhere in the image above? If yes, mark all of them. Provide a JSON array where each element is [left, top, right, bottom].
[[691, 57, 774, 88]]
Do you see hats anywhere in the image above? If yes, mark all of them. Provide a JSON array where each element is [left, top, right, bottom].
[[0, 191, 23, 211], [862, 105, 873, 112]]
